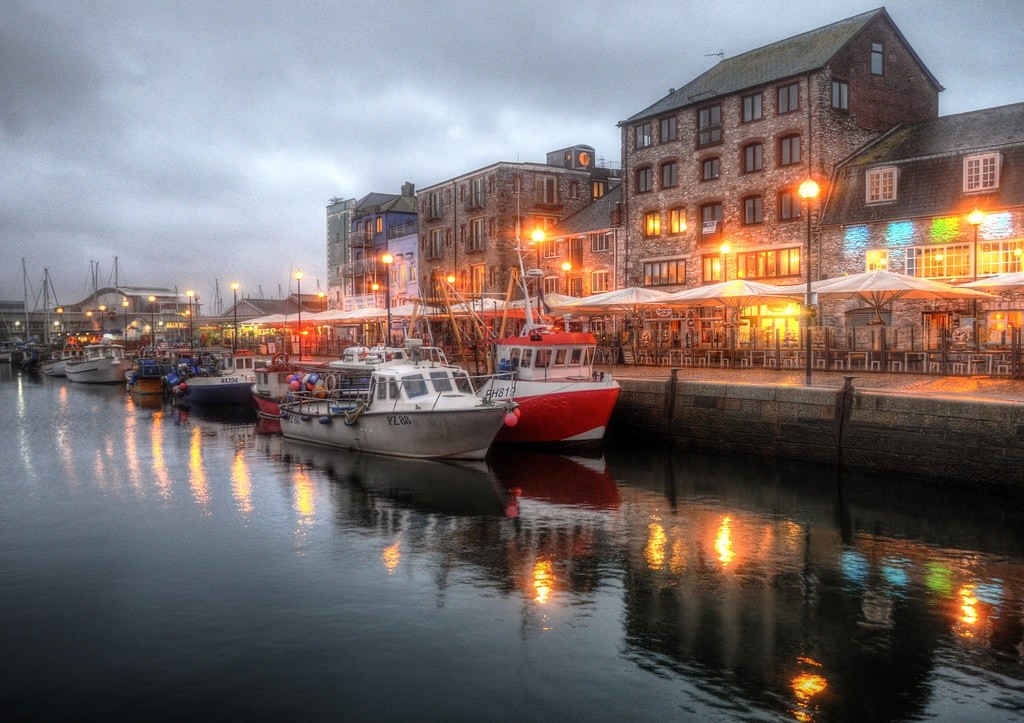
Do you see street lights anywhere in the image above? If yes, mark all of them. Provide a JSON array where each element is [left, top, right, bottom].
[[447, 272, 455, 308], [562, 260, 571, 295], [382, 252, 394, 345], [793, 523, 827, 697], [99, 304, 104, 333], [720, 242, 730, 349], [57, 307, 63, 325], [186, 288, 195, 350], [87, 311, 92, 324], [231, 279, 240, 349], [967, 206, 982, 350], [318, 290, 324, 349], [122, 298, 129, 341], [149, 295, 155, 351], [372, 282, 379, 309], [532, 225, 547, 323], [294, 268, 303, 360], [797, 175, 819, 383]]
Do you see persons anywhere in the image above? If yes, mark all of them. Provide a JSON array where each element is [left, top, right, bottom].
[[200, 332, 207, 350], [499, 359, 513, 380]]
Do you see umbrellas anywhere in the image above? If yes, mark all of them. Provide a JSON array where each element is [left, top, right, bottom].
[[487, 291, 581, 316], [767, 270, 1001, 349], [240, 308, 377, 353], [560, 285, 671, 345], [950, 271, 1024, 346], [643, 279, 802, 347], [445, 297, 511, 338], [374, 302, 443, 317]]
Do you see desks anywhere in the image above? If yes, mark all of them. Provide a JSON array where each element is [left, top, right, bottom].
[[795, 348, 846, 369], [750, 349, 794, 367], [707, 349, 748, 368], [904, 351, 948, 373], [848, 350, 891, 371], [669, 348, 707, 366], [965, 351, 1012, 375]]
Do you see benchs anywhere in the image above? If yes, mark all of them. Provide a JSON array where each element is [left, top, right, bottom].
[[601, 354, 1019, 374]]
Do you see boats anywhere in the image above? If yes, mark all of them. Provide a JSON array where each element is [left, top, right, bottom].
[[22, 347, 43, 372], [123, 358, 167, 394], [473, 236, 623, 439], [268, 437, 514, 516], [251, 365, 374, 436], [64, 335, 128, 384], [38, 335, 83, 376], [488, 449, 621, 508], [165, 351, 267, 408], [278, 338, 519, 458]]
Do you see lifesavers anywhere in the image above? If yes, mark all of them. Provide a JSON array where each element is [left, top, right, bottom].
[[236, 349, 249, 356], [271, 352, 290, 365]]
